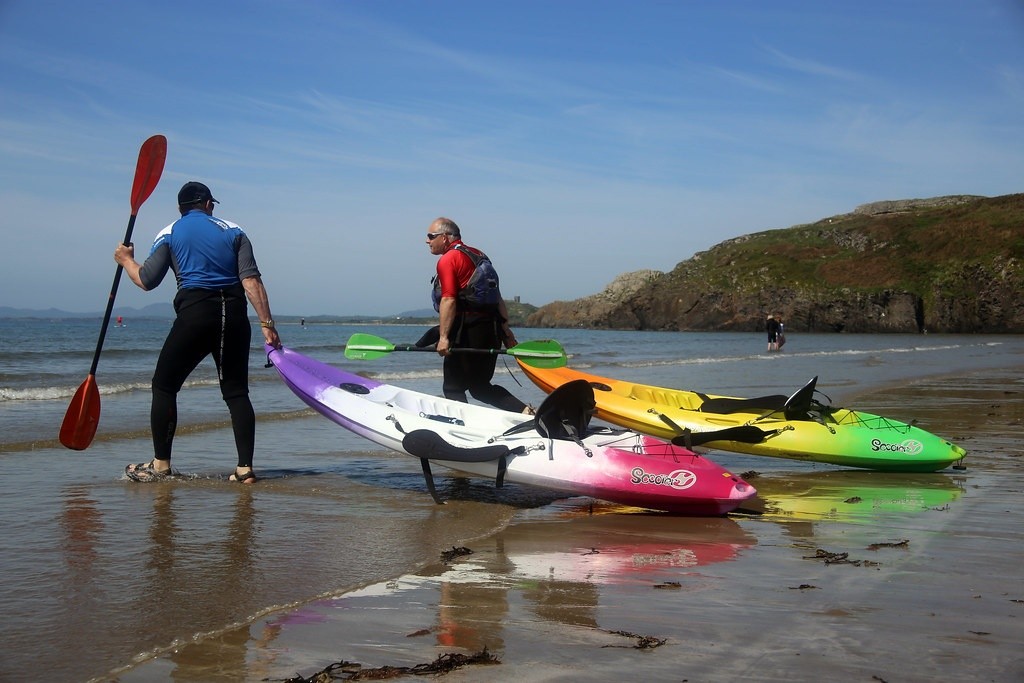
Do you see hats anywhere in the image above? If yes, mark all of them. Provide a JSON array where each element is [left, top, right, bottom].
[[767, 315, 774, 319], [178, 182, 221, 204]]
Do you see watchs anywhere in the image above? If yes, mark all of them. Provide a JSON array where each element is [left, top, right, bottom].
[[260, 319, 274, 328]]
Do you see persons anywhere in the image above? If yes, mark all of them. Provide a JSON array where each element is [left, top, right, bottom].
[[766, 314, 786, 351], [426, 217, 536, 417], [114, 182, 282, 485], [301, 318, 305, 327]]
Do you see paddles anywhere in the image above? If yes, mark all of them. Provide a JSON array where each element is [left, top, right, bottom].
[[343, 333, 567, 369], [58, 134, 167, 451]]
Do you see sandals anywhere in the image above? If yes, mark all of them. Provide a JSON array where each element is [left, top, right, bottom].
[[222, 469, 255, 483], [125, 459, 171, 482]]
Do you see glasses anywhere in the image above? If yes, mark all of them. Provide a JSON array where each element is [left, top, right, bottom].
[[428, 232, 451, 240]]
